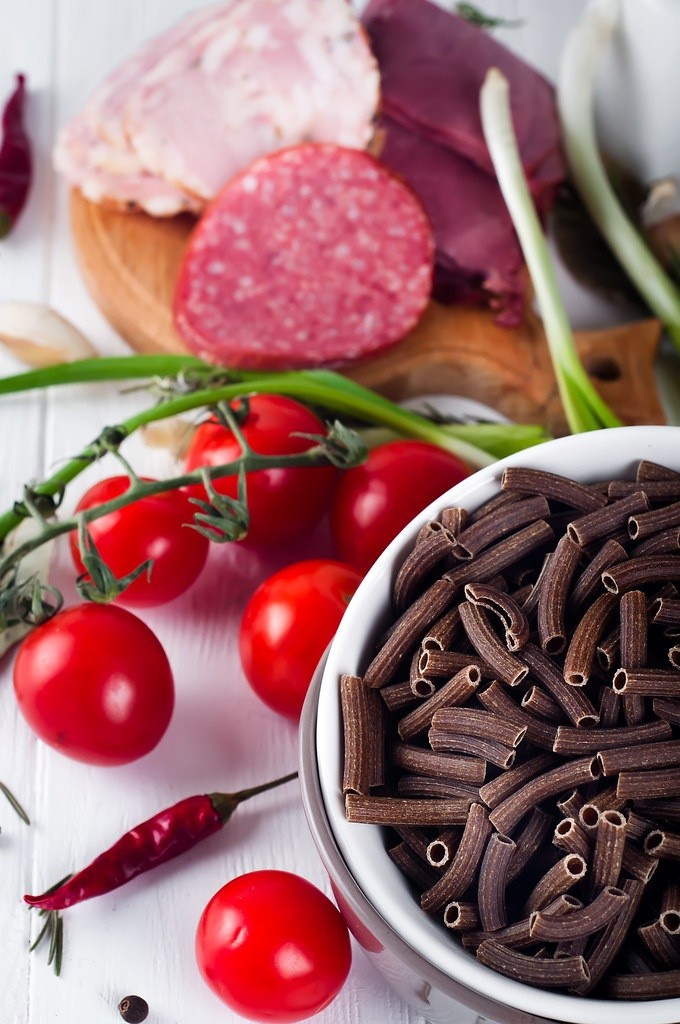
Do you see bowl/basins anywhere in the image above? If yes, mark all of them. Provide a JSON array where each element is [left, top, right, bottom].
[[316, 422, 678, 1024]]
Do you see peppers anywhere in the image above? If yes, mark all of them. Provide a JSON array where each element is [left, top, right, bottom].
[[23, 772, 302, 913]]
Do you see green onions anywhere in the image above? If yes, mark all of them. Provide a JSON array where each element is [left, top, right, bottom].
[[478, 27, 680, 434]]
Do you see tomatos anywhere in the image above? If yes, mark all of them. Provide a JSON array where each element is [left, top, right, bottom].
[[194, 871, 350, 1023], [325, 423, 478, 571], [183, 390, 335, 552], [237, 556, 365, 724], [12, 570, 177, 767], [69, 476, 211, 607]]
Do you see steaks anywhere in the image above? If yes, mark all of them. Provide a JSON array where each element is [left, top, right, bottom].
[[173, 144, 437, 373]]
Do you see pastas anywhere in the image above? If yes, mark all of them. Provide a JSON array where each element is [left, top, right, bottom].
[[338, 457, 679, 1003]]
